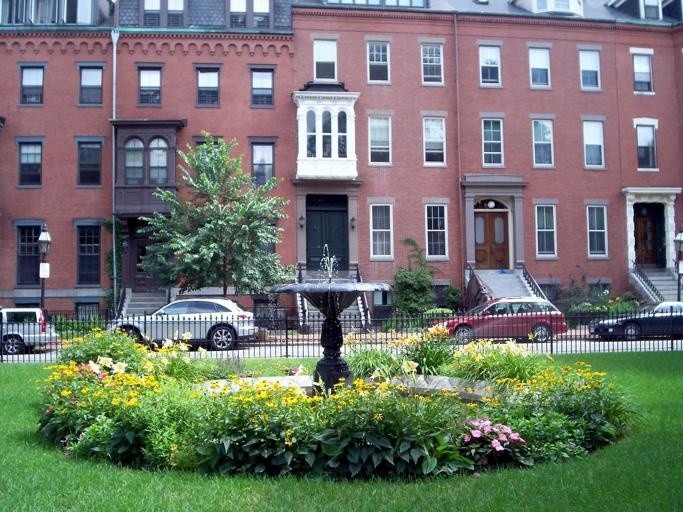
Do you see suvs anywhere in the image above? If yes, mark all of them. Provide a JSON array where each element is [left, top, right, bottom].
[[104, 295, 257, 350], [0, 305, 60, 355]]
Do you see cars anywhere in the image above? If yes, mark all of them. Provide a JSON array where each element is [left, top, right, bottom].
[[428, 296, 567, 346], [587, 300, 683, 341]]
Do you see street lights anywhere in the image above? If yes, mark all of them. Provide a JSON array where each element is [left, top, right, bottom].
[[672, 229, 683, 302], [37, 223, 51, 309]]
